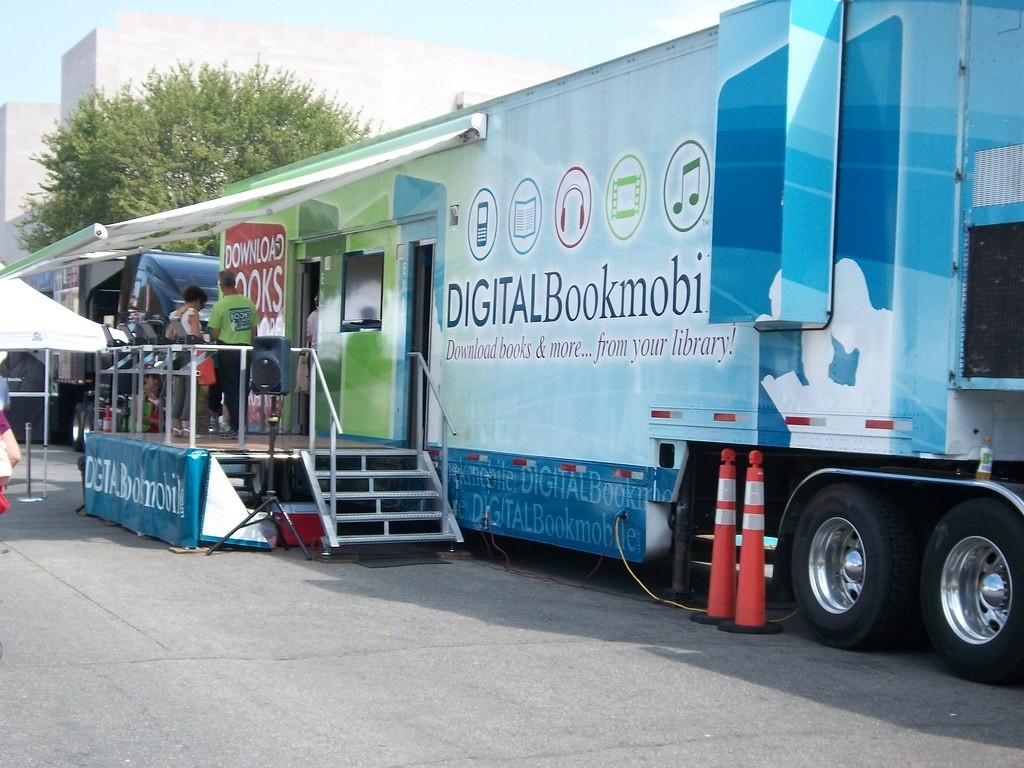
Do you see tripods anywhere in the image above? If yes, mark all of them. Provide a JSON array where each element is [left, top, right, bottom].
[[205, 396, 313, 562]]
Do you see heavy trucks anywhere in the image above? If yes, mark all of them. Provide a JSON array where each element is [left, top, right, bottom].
[[216, 0, 1024, 688], [0, 249, 221, 452]]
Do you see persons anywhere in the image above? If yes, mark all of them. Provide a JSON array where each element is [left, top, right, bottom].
[[0, 375, 21, 492], [139, 268, 259, 440], [306, 294, 319, 373]]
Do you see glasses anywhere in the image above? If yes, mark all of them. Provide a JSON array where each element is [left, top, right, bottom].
[[200, 303, 205, 309]]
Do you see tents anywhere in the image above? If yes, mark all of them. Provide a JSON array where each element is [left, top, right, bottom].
[[0, 278, 136, 499]]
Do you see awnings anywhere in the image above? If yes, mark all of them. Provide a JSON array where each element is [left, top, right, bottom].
[[0, 113, 488, 278]]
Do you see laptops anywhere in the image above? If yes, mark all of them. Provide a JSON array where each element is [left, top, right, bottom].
[[101, 318, 211, 346]]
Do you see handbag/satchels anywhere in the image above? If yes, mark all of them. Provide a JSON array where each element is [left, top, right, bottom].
[[294, 339, 310, 394], [0, 441, 12, 486]]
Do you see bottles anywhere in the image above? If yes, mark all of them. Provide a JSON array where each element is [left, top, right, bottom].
[[975, 435, 993, 480], [103, 406, 112, 432]]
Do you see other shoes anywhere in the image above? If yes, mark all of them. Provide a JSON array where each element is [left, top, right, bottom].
[[220, 428, 238, 438], [212, 419, 219, 434], [222, 422, 232, 431]]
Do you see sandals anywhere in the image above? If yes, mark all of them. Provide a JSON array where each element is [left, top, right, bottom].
[[172, 428, 183, 438], [183, 429, 201, 439]]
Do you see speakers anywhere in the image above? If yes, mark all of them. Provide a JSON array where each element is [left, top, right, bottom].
[[251, 336, 292, 396], [97, 353, 133, 399]]
